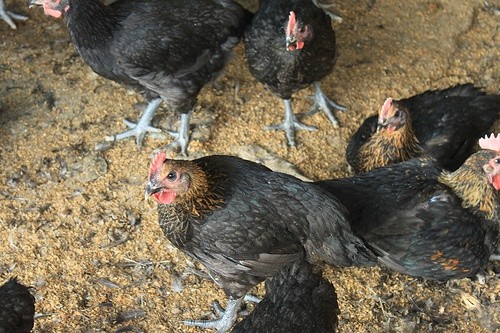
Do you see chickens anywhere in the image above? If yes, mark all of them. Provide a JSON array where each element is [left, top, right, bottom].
[[345, 82, 500, 178], [0, 0, 27, 31], [143, 152, 385, 332], [0, 277, 37, 333], [305, 132, 500, 285], [25, 1, 347, 156], [230, 262, 340, 333]]
